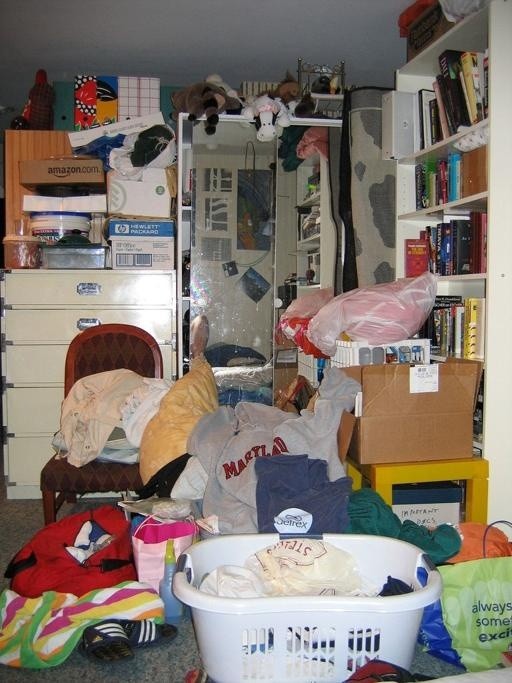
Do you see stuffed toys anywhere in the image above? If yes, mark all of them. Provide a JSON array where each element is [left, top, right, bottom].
[[168, 70, 343, 173]]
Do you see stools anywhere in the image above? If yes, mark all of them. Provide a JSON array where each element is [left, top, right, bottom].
[[346, 454, 490, 532]]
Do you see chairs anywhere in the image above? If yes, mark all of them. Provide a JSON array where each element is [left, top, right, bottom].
[[39, 322, 164, 527]]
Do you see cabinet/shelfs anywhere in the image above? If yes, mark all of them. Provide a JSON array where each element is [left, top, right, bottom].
[[1, 268, 178, 502], [393, 1, 512, 535], [295, 153, 335, 393]]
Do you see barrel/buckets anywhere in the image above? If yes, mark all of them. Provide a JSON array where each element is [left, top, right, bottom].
[[30, 211, 93, 268]]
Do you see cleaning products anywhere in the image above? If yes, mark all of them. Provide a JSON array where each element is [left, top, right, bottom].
[[158, 538, 186, 625]]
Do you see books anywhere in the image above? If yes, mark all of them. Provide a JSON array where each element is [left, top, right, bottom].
[[405, 42, 492, 442]]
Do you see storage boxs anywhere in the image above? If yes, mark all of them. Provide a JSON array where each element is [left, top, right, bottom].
[[17, 150, 176, 268], [406, 2, 457, 63], [308, 355, 484, 536]]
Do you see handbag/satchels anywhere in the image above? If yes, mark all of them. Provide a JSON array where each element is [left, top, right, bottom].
[[128, 509, 201, 598], [434, 516, 512, 675]]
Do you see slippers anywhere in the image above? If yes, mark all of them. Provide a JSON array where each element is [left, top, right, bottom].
[[76, 619, 135, 666], [119, 619, 178, 650]]
